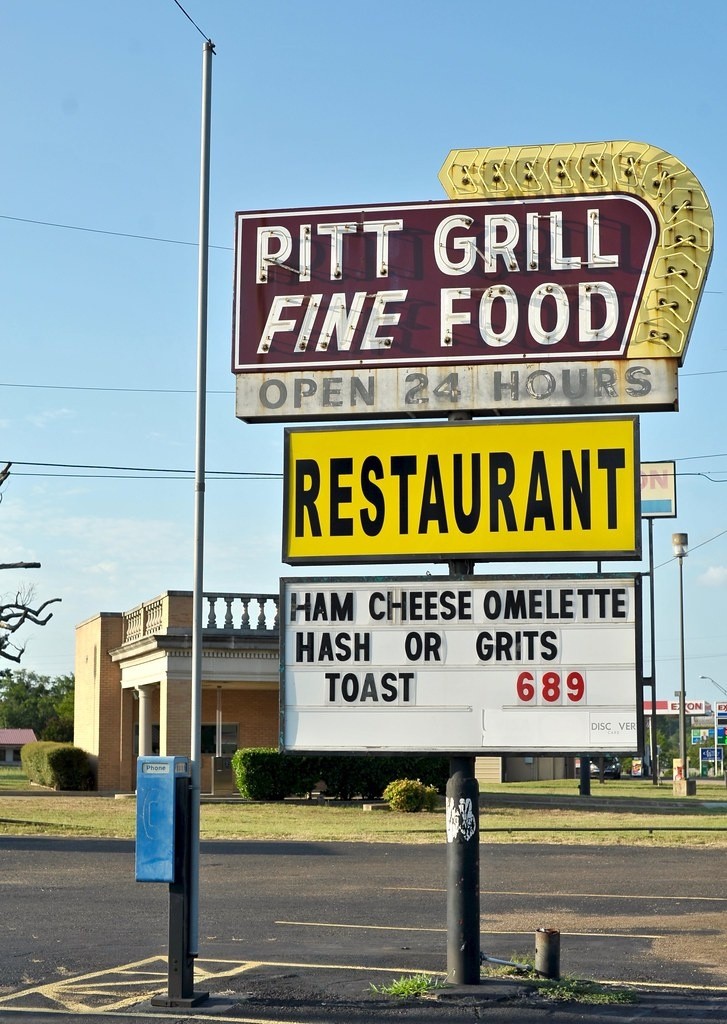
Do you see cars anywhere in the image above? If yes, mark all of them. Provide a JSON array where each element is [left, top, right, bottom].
[[659, 762, 672, 779], [588, 756, 624, 780]]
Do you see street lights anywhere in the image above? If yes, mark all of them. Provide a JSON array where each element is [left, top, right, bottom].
[[700, 675, 727, 696], [671, 532, 697, 798]]
